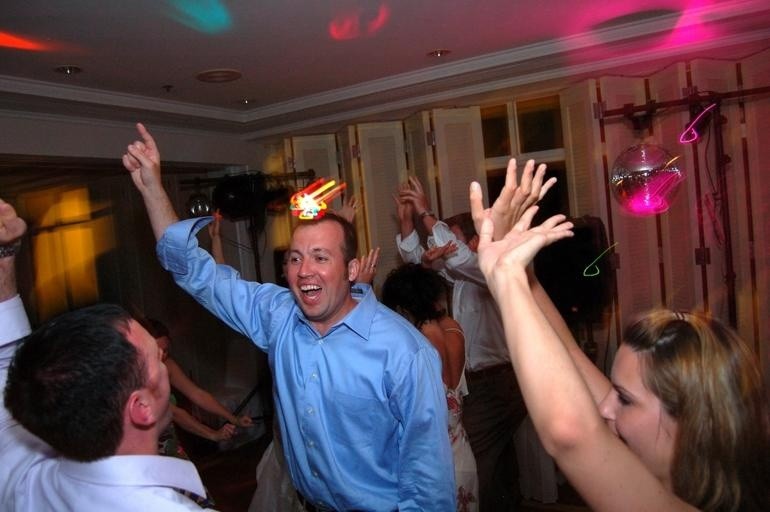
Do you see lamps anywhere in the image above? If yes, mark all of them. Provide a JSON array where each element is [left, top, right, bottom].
[[611, 143, 687, 216]]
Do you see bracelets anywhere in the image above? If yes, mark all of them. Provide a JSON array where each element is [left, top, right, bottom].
[[416, 211, 435, 218], [0, 238, 22, 259]]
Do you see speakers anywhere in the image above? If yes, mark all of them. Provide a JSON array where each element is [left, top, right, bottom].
[[216, 175, 265, 217], [534, 216, 613, 320]]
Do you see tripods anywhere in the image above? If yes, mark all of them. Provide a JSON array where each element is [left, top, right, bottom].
[[222, 218, 275, 427]]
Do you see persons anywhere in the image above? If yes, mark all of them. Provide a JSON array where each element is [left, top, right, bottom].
[[388, 175, 535, 512], [0, 192, 225, 512], [120, 120, 461, 511], [132, 311, 255, 511], [469, 156, 768, 509], [348, 246, 482, 511]]
